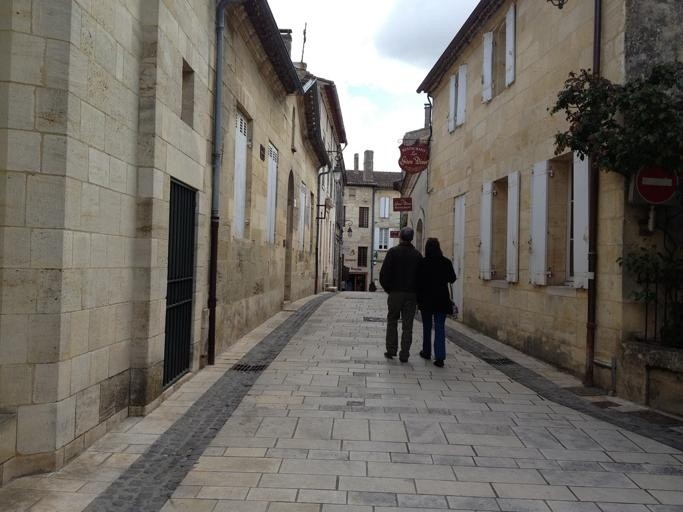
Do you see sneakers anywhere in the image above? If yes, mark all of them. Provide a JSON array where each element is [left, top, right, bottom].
[[420, 351, 430, 359], [384, 352, 396, 356], [400, 355, 408, 361], [434, 361, 444, 366]]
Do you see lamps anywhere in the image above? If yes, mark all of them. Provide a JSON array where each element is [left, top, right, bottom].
[[327, 150, 342, 182], [338, 219, 353, 239]]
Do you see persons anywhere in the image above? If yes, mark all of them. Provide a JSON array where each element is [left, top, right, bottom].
[[414, 238, 457, 367], [379, 227, 424, 362]]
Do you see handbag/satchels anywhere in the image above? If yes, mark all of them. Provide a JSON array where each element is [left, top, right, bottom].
[[449, 298, 458, 315]]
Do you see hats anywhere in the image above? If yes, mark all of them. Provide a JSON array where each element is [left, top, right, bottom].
[[401, 228, 413, 240]]
